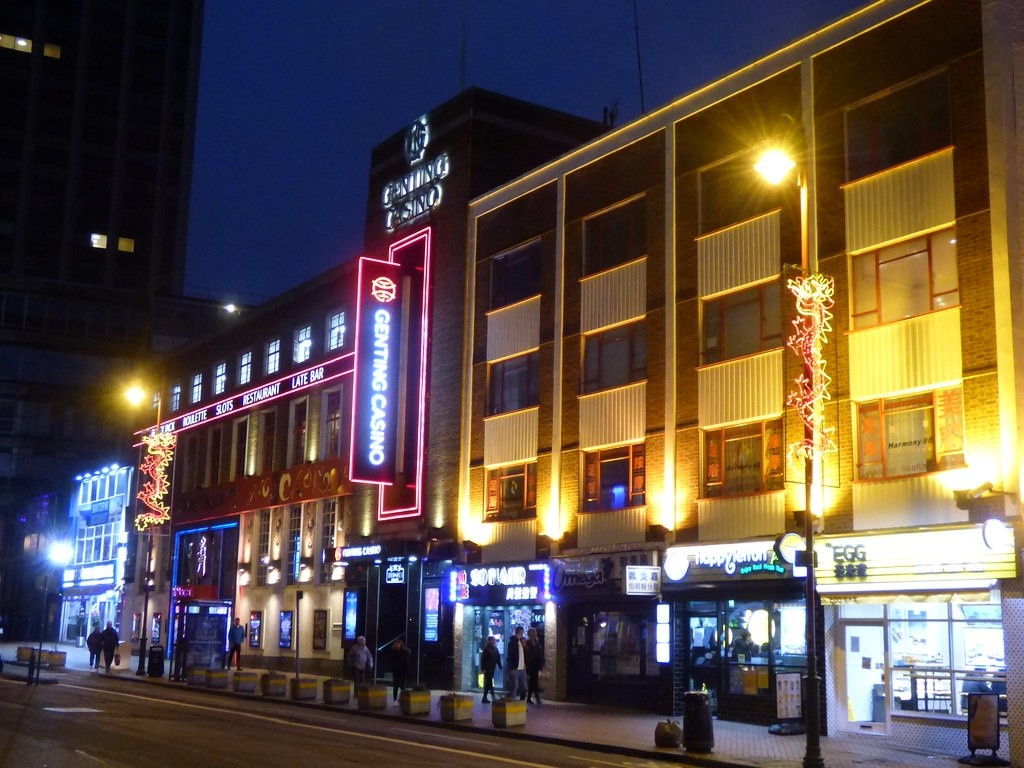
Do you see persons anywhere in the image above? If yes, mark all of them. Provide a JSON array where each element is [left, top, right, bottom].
[[480, 637, 503, 703], [348, 636, 373, 698], [87, 620, 119, 673], [732, 630, 769, 658], [507, 626, 544, 705], [387, 640, 410, 706], [960, 665, 1007, 709], [228, 617, 244, 671]]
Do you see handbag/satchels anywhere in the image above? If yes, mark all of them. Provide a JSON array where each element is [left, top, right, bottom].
[[115, 653, 121, 665]]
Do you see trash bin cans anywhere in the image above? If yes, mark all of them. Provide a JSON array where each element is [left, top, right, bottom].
[[147, 645, 164, 677], [683, 691, 714, 752]]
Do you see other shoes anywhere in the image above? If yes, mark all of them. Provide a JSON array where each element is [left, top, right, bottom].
[[106, 667, 110, 673], [394, 701, 397, 706], [228, 665, 231, 670], [94, 668, 98, 673], [89, 665, 92, 670], [482, 699, 491, 703], [237, 667, 243, 670]]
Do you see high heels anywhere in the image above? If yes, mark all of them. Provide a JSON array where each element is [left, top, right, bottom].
[[527, 698, 534, 704], [536, 699, 542, 704]]
